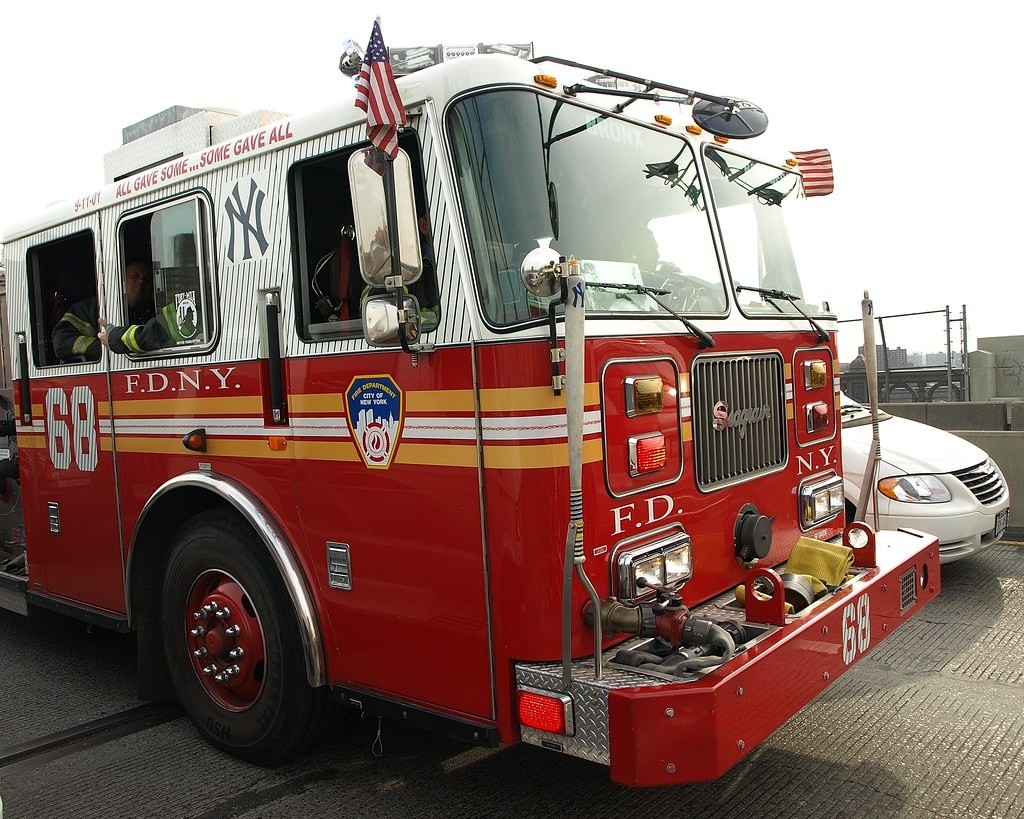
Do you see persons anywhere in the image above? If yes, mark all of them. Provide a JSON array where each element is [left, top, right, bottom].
[[624, 228, 660, 271], [412, 209, 437, 309], [544, 266, 555, 294], [97, 302, 194, 355], [51, 262, 153, 364]]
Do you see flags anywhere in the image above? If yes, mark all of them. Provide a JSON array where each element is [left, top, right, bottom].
[[793, 149, 834, 197], [355, 20, 407, 159]]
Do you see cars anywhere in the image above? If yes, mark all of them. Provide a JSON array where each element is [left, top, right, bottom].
[[838, 389, 1012, 566]]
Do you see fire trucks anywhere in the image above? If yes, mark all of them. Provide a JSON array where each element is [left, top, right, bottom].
[[0, 16, 945, 796]]
